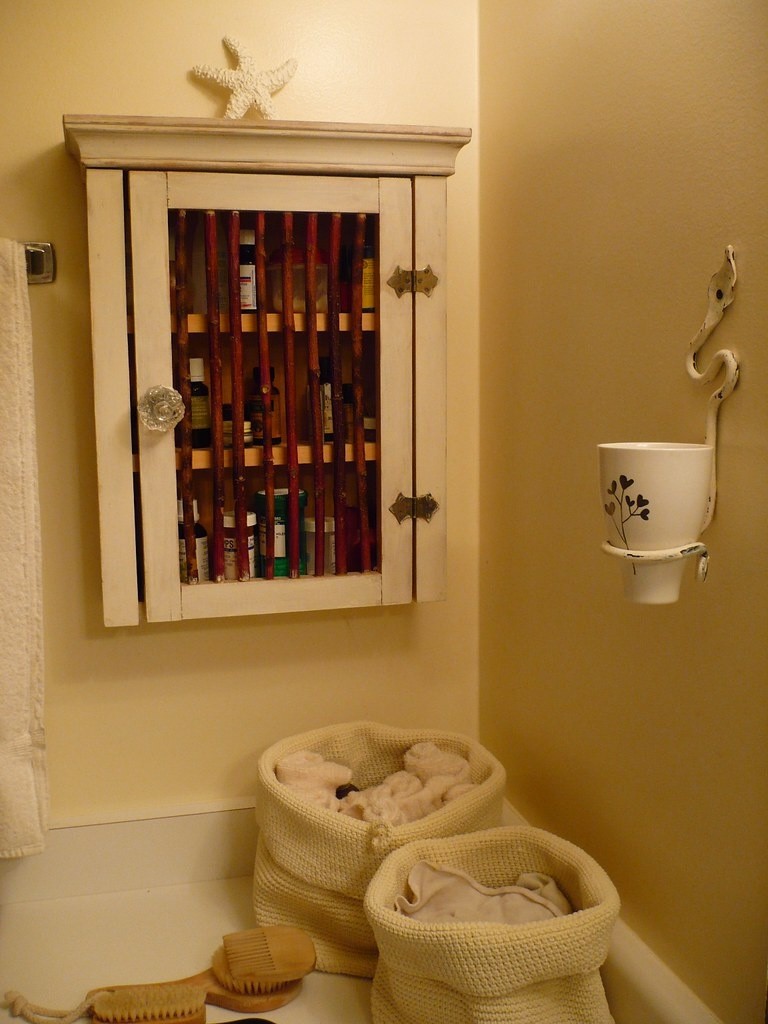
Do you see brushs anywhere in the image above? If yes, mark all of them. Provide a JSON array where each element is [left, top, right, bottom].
[[83, 940, 304, 1024]]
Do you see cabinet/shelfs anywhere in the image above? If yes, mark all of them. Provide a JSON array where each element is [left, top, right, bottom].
[[62, 112, 475, 630]]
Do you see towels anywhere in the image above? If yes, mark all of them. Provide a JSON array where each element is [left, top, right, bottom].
[[277, 742, 480, 825], [1, 240, 55, 862]]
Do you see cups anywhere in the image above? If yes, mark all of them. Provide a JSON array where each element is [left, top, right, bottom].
[[598, 442, 714, 604]]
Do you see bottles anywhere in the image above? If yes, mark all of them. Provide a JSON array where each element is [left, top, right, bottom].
[[362, 236, 375, 313], [343, 383, 356, 444], [307, 358, 333, 444], [249, 367, 281, 445], [244, 421, 253, 447], [255, 489, 308, 578], [363, 382, 377, 442], [222, 511, 257, 581], [176, 498, 209, 582], [240, 229, 258, 314], [189, 358, 209, 449], [303, 515, 337, 575], [222, 404, 233, 448]]
